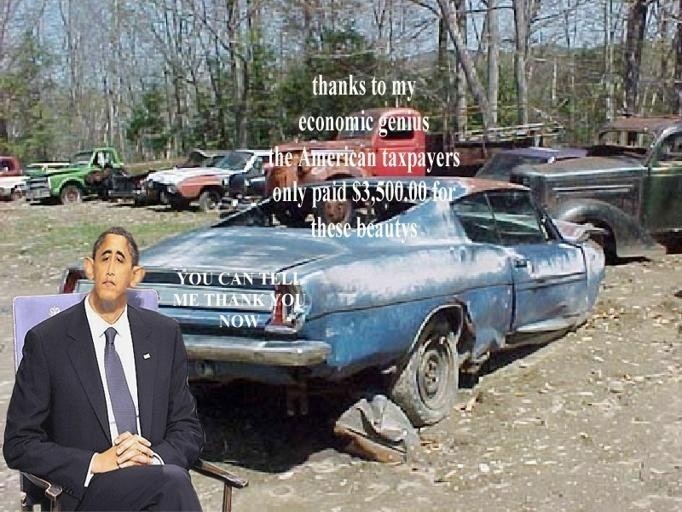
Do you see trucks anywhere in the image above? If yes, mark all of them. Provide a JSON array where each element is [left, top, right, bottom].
[[264, 99, 556, 230]]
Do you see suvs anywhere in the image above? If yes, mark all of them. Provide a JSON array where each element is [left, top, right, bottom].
[[509, 114, 682, 258]]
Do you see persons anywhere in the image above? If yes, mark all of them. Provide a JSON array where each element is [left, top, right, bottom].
[[3, 226, 208, 512]]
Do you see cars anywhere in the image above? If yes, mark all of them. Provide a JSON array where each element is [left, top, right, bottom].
[[57, 172, 608, 433], [0, 144, 277, 213], [466, 141, 586, 203]]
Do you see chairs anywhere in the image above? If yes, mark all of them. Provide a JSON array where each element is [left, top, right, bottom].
[[11, 286, 250, 512]]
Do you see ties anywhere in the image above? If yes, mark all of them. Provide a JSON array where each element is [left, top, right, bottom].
[[101, 324, 138, 440]]
